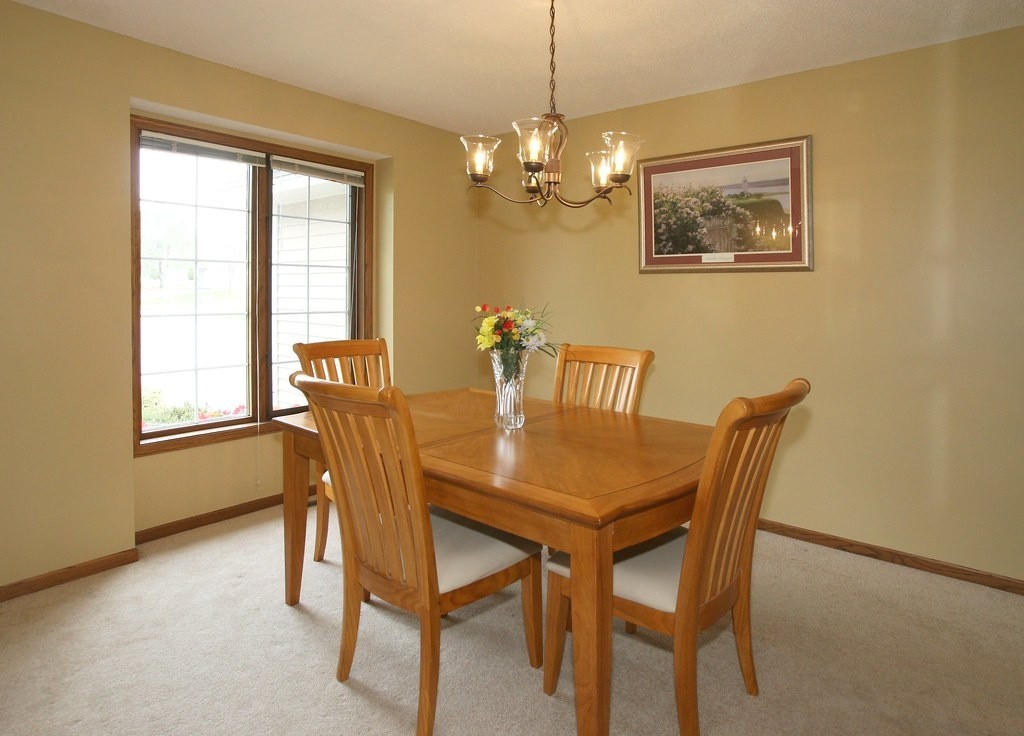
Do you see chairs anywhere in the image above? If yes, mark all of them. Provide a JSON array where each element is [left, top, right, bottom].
[[545, 378, 811, 736], [290, 370, 544, 736], [293, 338, 392, 603], [551, 345, 655, 636]]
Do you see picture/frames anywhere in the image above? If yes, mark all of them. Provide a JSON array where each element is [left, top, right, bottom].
[[636, 135, 815, 275]]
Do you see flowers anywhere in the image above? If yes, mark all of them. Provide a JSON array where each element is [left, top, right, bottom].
[[470, 297, 570, 389]]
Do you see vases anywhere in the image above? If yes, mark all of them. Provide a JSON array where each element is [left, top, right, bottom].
[[487, 348, 529, 430]]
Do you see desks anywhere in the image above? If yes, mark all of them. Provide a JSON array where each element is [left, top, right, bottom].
[[269, 388, 716, 736]]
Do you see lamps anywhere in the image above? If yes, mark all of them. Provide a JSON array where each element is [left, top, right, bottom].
[[460, 1, 647, 209]]
